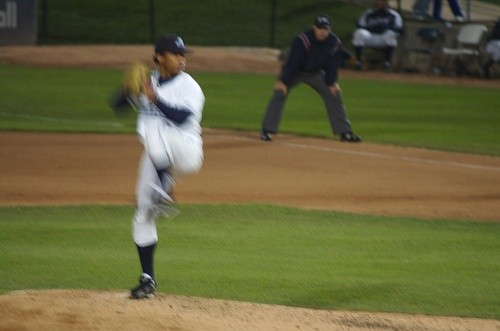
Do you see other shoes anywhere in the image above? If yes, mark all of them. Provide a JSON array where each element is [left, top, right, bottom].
[[341, 131, 361, 142], [260, 131, 273, 141], [383, 63, 391, 71], [354, 63, 361, 70], [455, 16, 464, 21]]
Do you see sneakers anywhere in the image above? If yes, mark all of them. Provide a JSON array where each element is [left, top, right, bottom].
[[131, 273, 156, 298], [149, 182, 175, 205]]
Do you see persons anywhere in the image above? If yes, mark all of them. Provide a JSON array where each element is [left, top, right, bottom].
[[413, 0, 465, 21], [260, 16, 360, 143], [111, 32, 207, 298], [351, 0, 404, 68]]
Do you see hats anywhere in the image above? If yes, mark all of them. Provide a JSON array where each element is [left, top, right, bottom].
[[156, 33, 193, 53], [313, 15, 332, 26]]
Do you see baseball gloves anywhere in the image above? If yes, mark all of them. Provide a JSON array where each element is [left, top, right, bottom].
[[124, 59, 151, 96]]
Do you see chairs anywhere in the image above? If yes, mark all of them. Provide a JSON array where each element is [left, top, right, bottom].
[[441, 24, 488, 77], [400, 26, 442, 75], [365, 45, 396, 71]]
